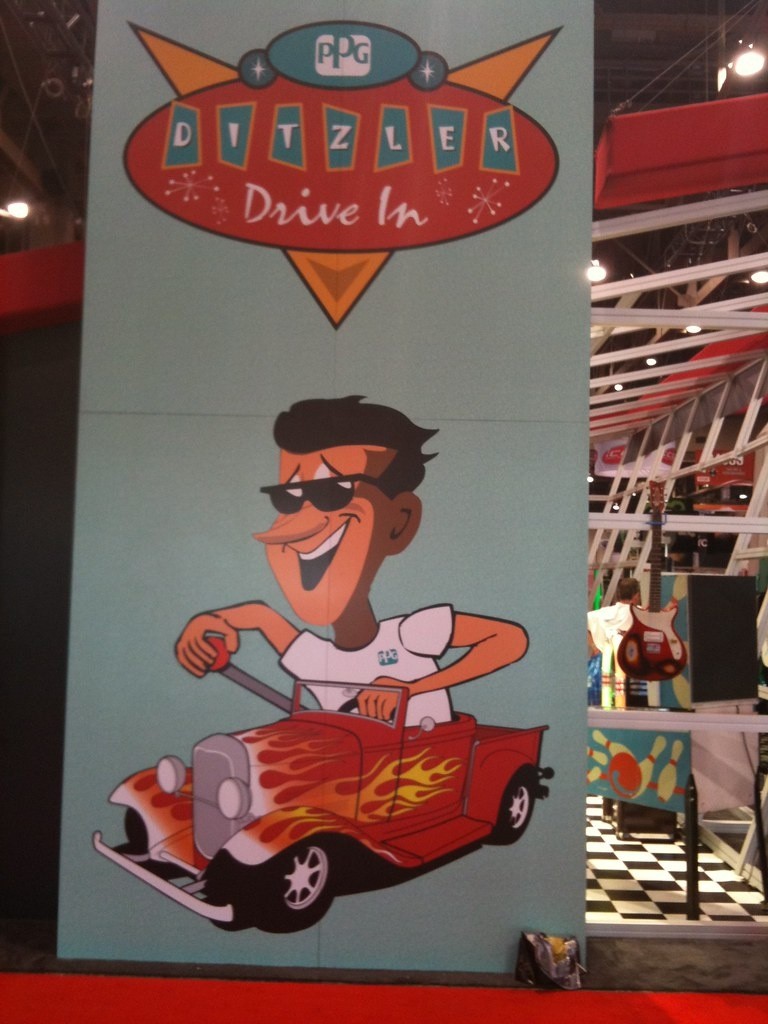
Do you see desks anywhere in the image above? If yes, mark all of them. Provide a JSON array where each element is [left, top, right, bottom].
[[588, 702, 768, 920]]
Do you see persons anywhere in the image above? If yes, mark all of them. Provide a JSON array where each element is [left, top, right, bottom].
[[617, 578, 641, 606]]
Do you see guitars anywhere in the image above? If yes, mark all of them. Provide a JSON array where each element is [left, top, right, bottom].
[[614, 477, 690, 683]]
